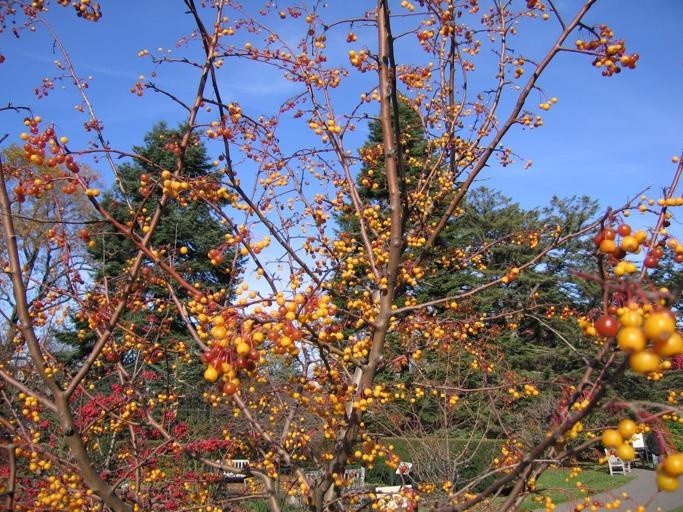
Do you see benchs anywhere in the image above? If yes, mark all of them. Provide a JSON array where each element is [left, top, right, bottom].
[[213, 459, 250, 487], [605, 449, 630, 476]]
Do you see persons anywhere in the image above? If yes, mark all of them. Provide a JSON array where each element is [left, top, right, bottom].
[[645, 430, 661, 470]]
